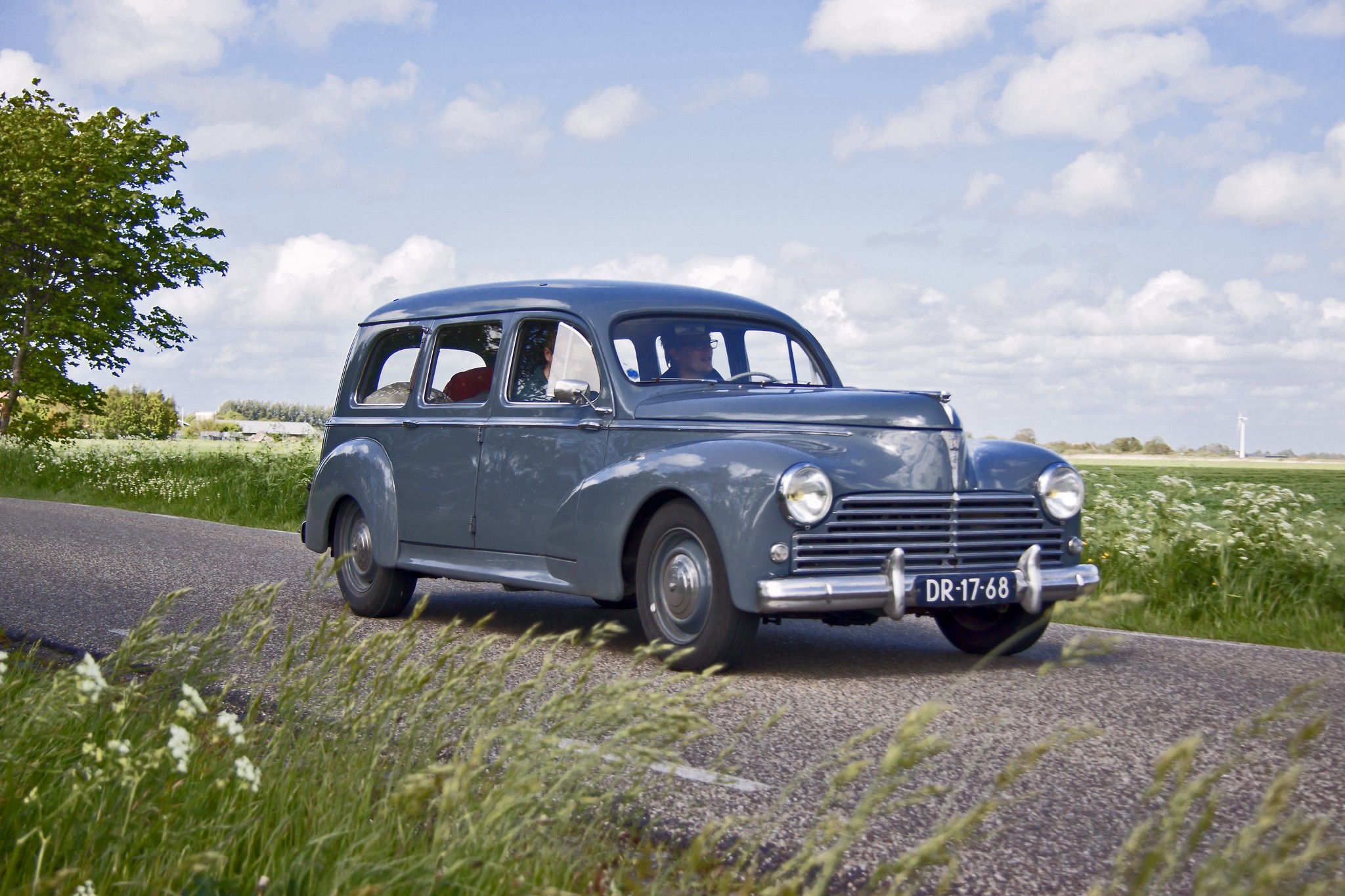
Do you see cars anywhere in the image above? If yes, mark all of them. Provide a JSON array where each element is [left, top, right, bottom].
[[299, 282, 1100, 673]]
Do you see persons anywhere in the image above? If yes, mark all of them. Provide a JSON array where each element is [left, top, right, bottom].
[[512, 330, 560, 402], [657, 320, 728, 383]]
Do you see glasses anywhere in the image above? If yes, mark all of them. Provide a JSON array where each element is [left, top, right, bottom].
[[675, 339, 718, 351]]
